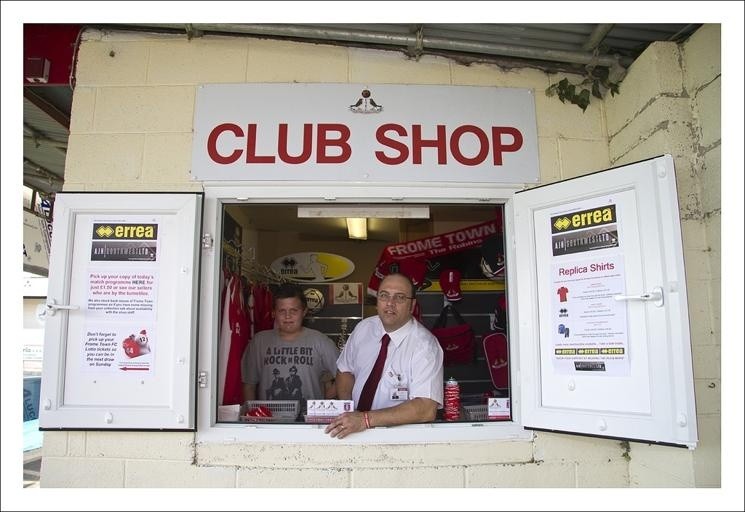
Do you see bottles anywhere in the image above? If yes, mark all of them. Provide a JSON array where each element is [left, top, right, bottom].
[[443, 376, 460, 418]]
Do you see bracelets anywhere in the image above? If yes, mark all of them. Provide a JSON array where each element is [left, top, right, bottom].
[[363, 410, 372, 431]]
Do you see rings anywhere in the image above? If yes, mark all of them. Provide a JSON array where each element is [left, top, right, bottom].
[[339, 424, 346, 430]]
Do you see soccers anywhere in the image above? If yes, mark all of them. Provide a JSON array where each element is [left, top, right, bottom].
[[302, 287, 325, 314]]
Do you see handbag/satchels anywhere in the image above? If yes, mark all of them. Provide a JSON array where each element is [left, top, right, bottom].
[[430, 305, 476, 368], [481, 314, 509, 390]]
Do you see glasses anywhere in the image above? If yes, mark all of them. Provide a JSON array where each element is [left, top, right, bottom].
[[376, 292, 416, 304]]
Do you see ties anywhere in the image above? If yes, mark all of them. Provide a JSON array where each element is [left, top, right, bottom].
[[357, 334, 391, 412]]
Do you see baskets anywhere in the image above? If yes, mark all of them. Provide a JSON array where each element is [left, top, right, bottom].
[[301, 411, 337, 424], [239, 399, 301, 423], [461, 405, 488, 421]]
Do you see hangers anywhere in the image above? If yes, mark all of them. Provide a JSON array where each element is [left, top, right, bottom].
[[222, 238, 287, 288]]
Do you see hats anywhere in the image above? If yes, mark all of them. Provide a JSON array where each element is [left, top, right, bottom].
[[439, 269, 465, 302], [425, 257, 458, 279]]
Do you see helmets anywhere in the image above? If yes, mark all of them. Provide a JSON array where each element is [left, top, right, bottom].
[[480, 254, 505, 281]]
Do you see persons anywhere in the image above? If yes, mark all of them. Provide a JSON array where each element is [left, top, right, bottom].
[[265, 367, 286, 400], [391, 390, 399, 400], [285, 365, 302, 399], [240, 283, 339, 420], [324, 274, 445, 439]]
